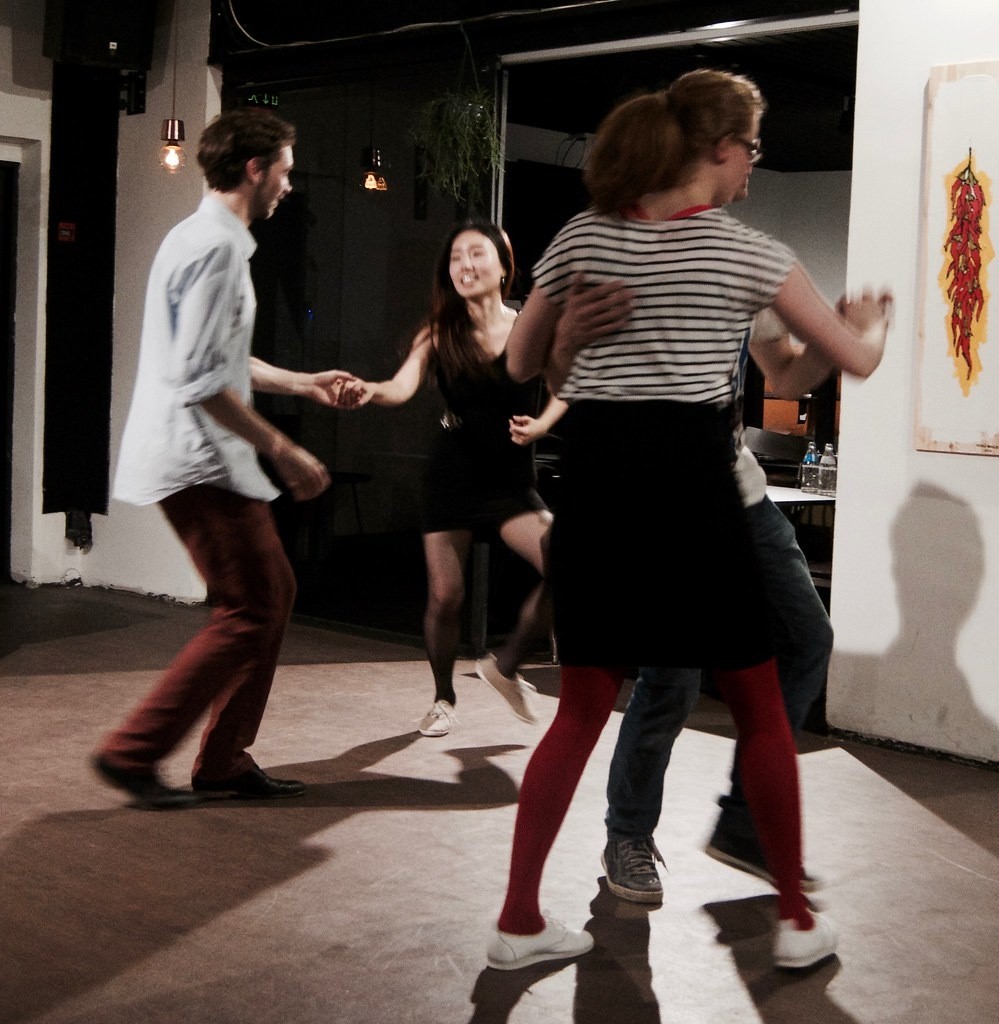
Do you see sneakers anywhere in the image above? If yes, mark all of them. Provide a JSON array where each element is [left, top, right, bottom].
[[474, 652, 540, 725], [772, 908, 840, 967], [418, 699, 462, 736], [705, 794, 826, 892], [486, 909, 595, 970], [600, 835, 668, 902]]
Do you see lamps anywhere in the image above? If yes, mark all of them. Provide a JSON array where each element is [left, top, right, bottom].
[[359, 144, 389, 191]]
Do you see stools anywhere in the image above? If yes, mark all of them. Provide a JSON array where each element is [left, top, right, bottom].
[[326, 471, 371, 542]]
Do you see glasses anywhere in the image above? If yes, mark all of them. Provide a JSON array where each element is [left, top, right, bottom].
[[712, 131, 762, 160]]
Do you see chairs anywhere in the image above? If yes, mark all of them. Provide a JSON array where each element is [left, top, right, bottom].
[[532, 431, 557, 479], [745, 426, 833, 589]]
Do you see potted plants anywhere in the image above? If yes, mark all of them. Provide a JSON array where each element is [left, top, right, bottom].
[[410, 86, 510, 211]]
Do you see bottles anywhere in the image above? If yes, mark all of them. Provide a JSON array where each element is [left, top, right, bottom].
[[819, 443, 837, 496], [801, 442, 819, 493]]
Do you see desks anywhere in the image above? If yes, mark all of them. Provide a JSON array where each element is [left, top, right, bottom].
[[765, 483, 836, 508]]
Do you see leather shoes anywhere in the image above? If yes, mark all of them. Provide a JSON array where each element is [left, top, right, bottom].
[[90, 754, 198, 805], [193, 764, 306, 800]]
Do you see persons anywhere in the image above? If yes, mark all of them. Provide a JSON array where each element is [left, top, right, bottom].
[[334, 219, 569, 735], [88, 100, 365, 805], [485, 67, 893, 972]]
